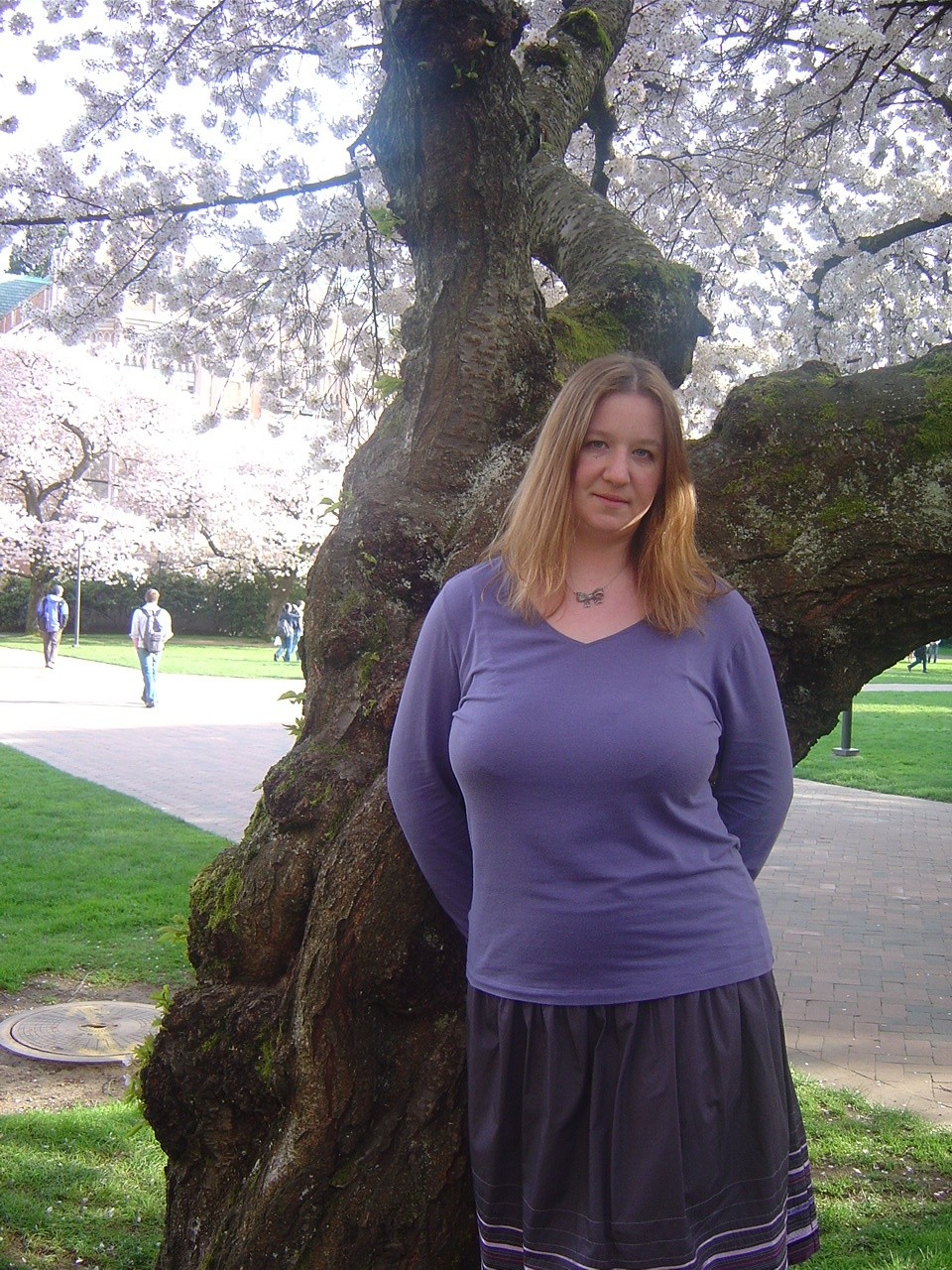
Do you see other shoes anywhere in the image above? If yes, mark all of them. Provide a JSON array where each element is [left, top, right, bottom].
[[923, 670, 929, 673], [298, 659, 302, 663], [283, 655, 290, 662], [274, 653, 279, 662], [907, 665, 911, 672], [45, 661, 55, 669], [141, 696, 155, 708]]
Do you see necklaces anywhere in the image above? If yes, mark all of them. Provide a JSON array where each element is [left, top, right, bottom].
[[562, 558, 631, 609]]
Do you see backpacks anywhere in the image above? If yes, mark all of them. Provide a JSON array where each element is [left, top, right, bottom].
[[277, 613, 293, 638], [137, 607, 165, 653]]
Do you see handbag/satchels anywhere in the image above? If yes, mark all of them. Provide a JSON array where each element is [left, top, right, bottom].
[[274, 635, 282, 647]]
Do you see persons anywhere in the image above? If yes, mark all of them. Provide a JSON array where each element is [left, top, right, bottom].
[[36, 584, 69, 669], [907, 642, 938, 673], [129, 588, 174, 707], [274, 599, 306, 661], [387, 351, 819, 1270]]
